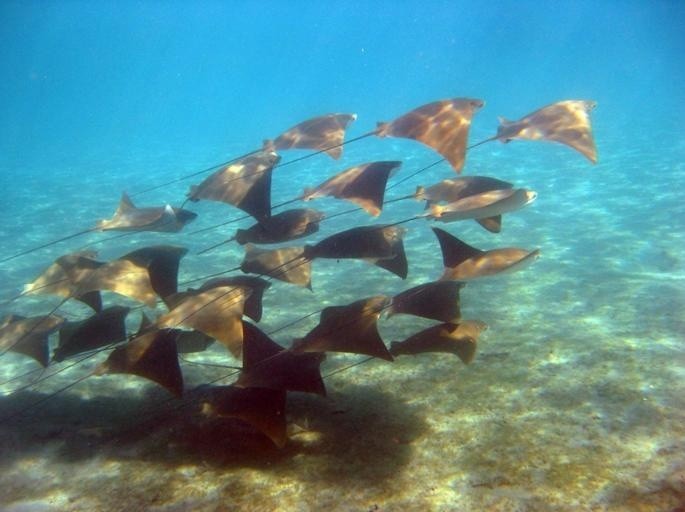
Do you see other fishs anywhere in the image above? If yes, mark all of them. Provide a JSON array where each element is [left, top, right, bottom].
[[0, 99, 597, 459]]
